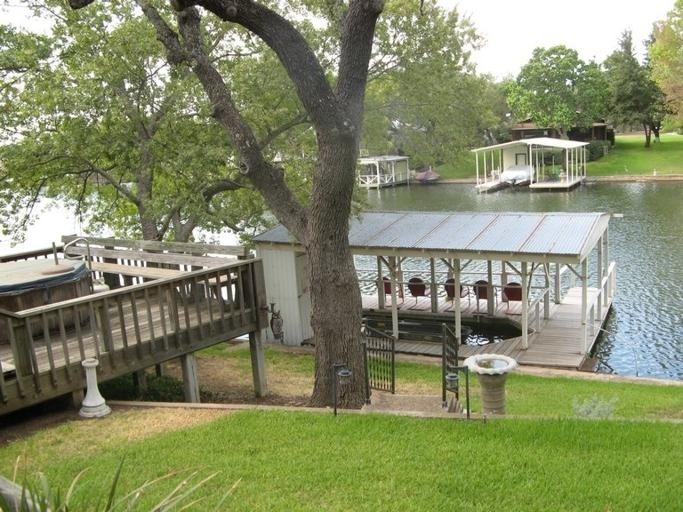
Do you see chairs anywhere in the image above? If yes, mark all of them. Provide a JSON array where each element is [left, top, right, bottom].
[[375, 277, 522, 315]]
